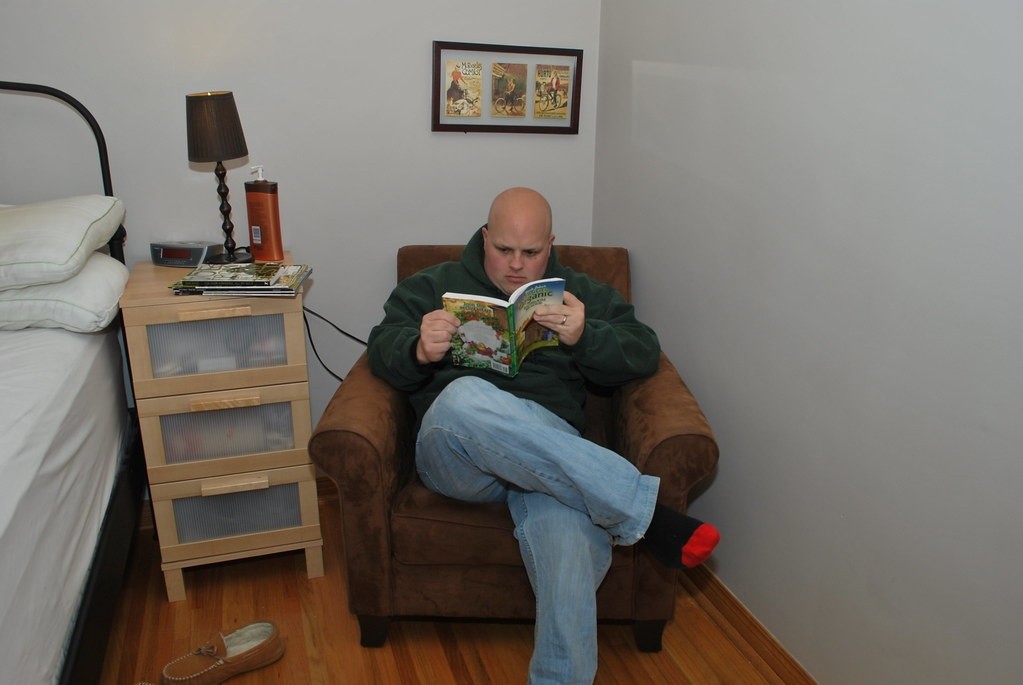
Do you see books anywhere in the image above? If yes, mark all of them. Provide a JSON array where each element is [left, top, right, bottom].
[[442, 278, 565, 377], [166, 263, 313, 297]]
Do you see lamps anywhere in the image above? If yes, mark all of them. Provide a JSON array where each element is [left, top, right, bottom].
[[184, 89, 251, 263]]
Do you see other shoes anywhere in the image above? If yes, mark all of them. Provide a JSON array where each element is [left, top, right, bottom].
[[510, 108, 513, 111], [553, 104, 557, 107], [159, 622, 284, 685]]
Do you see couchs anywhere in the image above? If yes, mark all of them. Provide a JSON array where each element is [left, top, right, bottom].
[[308, 243, 723, 655]]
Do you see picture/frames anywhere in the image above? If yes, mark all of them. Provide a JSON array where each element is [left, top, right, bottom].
[[432, 41, 583, 134]]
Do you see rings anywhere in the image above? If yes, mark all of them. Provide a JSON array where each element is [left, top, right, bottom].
[[562, 316, 566, 325]]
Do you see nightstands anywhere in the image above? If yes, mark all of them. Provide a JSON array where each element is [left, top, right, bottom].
[[117, 255, 325, 608]]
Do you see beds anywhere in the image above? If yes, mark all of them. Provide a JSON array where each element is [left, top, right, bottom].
[[0, 82, 153, 685]]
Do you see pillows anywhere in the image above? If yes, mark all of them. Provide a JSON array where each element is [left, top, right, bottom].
[[0, 195, 132, 336]]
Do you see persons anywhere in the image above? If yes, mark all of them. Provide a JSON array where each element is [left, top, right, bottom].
[[502, 78, 515, 110], [367, 188, 721, 685], [547, 70, 560, 108], [447, 65, 469, 103]]
